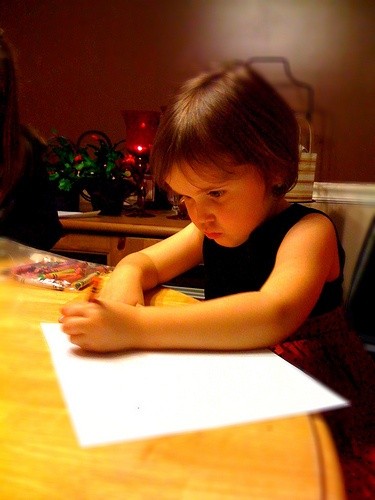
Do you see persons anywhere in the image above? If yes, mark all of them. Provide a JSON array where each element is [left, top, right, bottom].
[[0, 30, 69, 252], [59, 64, 375, 500]]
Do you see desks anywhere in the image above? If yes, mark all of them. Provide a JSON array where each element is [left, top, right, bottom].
[[0, 252, 346, 500], [59, 209, 193, 267]]
